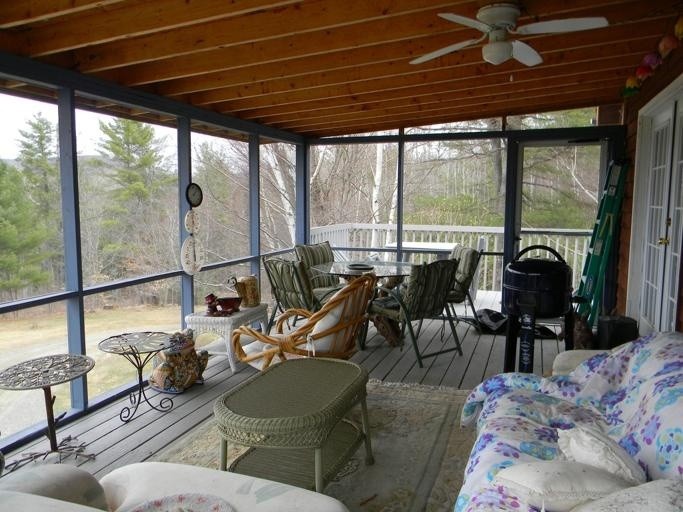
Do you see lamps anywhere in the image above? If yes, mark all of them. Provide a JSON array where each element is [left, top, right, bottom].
[[482, 31, 512, 65]]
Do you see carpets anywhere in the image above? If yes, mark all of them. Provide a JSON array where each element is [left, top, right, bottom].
[[156, 379, 477, 512]]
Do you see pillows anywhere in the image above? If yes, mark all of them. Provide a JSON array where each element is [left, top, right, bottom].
[[571, 477, 683, 511], [493, 462, 630, 511], [557, 427, 646, 486]]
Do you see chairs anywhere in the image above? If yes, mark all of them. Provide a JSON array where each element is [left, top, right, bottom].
[[0, 462, 349, 512], [231, 235, 486, 371]]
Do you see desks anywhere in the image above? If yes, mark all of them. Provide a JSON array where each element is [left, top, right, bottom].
[[185, 304, 268, 373], [0, 354, 96, 478], [98, 331, 180, 422]]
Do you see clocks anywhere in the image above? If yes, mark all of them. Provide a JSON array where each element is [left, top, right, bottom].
[[181, 236, 204, 275], [186, 183, 204, 207], [185, 210, 201, 233]]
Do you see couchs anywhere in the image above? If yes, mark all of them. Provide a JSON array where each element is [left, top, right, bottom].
[[454, 333, 683, 511]]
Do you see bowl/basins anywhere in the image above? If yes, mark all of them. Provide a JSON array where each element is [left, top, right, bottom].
[[218, 297, 242, 313]]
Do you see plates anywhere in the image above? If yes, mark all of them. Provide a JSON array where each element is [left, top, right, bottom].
[[347, 263, 375, 270]]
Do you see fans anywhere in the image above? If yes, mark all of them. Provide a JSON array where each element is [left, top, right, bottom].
[[408, 4, 609, 67]]
